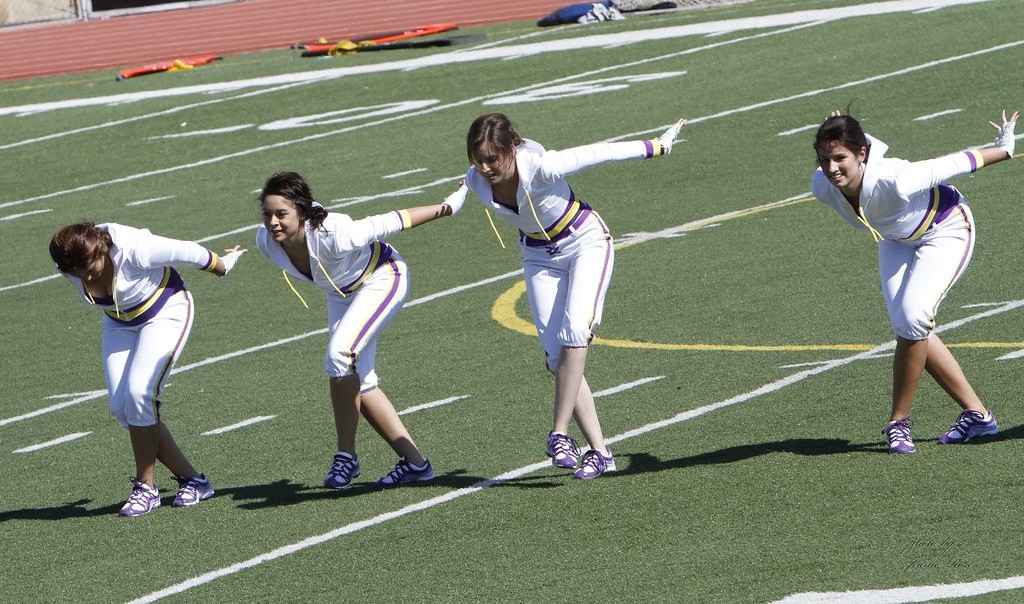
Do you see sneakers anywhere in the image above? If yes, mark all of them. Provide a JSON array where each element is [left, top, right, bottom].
[[379, 458, 434, 485], [171, 472, 215, 506], [545, 431, 582, 468], [120, 477, 162, 517], [881, 417, 916, 453], [939, 409, 999, 444], [324, 451, 360, 488], [574, 448, 616, 480]]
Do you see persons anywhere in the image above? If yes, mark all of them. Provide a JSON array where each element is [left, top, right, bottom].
[[812, 95, 1021, 453], [464, 113, 687, 480], [257, 171, 467, 489], [50, 219, 249, 518]]
